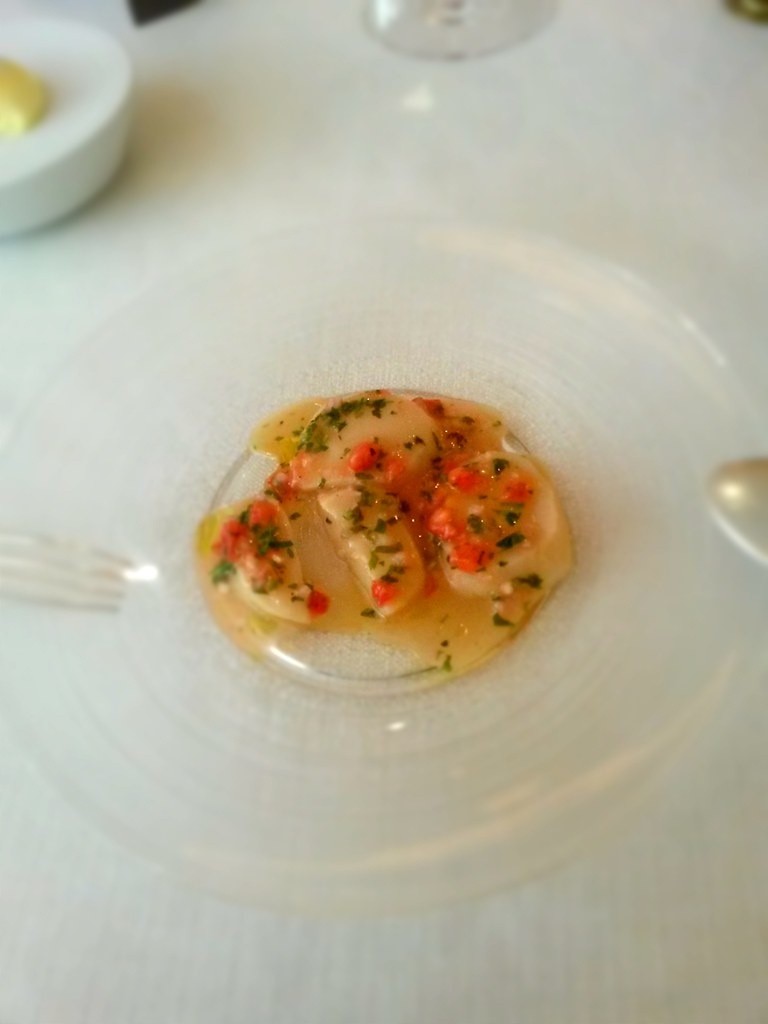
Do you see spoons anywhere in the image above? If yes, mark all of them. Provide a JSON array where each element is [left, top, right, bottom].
[[705, 454, 766, 568]]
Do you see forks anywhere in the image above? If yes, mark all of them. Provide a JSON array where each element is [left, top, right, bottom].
[[1, 534, 137, 613]]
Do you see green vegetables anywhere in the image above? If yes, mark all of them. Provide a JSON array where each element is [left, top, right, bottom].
[[205, 392, 544, 675]]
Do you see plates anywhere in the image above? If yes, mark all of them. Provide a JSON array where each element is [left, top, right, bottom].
[[0, 0, 768, 1022]]
